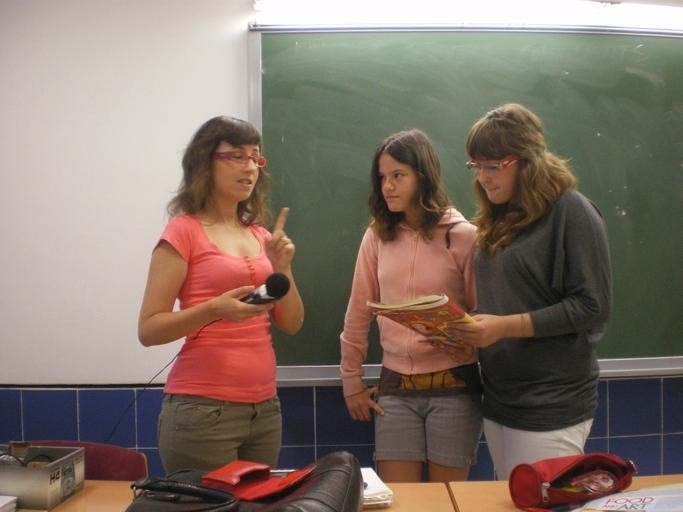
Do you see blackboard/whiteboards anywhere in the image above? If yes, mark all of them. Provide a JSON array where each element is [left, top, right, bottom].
[[249, 25, 683, 387]]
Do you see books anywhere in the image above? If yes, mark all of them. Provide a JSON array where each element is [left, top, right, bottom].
[[366, 294, 476, 348]]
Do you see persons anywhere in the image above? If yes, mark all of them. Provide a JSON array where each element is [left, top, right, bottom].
[[137, 115, 305, 475], [338, 127, 480, 482], [443, 103, 612, 481]]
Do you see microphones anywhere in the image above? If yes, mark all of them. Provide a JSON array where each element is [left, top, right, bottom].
[[239, 273, 290, 305]]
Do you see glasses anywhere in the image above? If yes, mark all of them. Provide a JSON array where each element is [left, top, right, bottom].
[[212, 149, 266, 170], [464, 157, 519, 173]]
